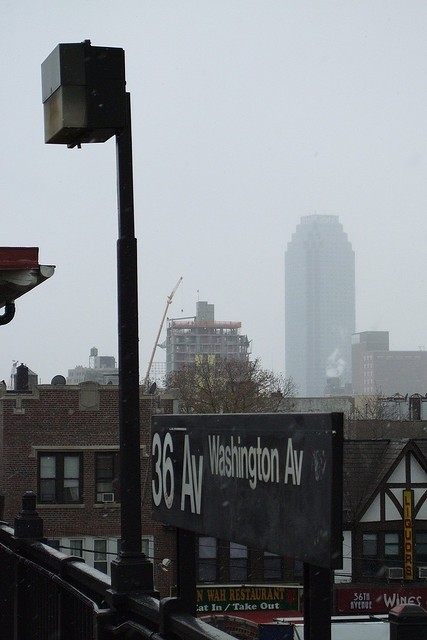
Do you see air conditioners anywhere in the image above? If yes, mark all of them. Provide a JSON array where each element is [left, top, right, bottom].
[[100, 492, 114, 503], [385, 567, 404, 580], [417, 567, 427, 579]]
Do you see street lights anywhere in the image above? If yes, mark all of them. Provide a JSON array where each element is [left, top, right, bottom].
[[38, 39, 155, 598]]
